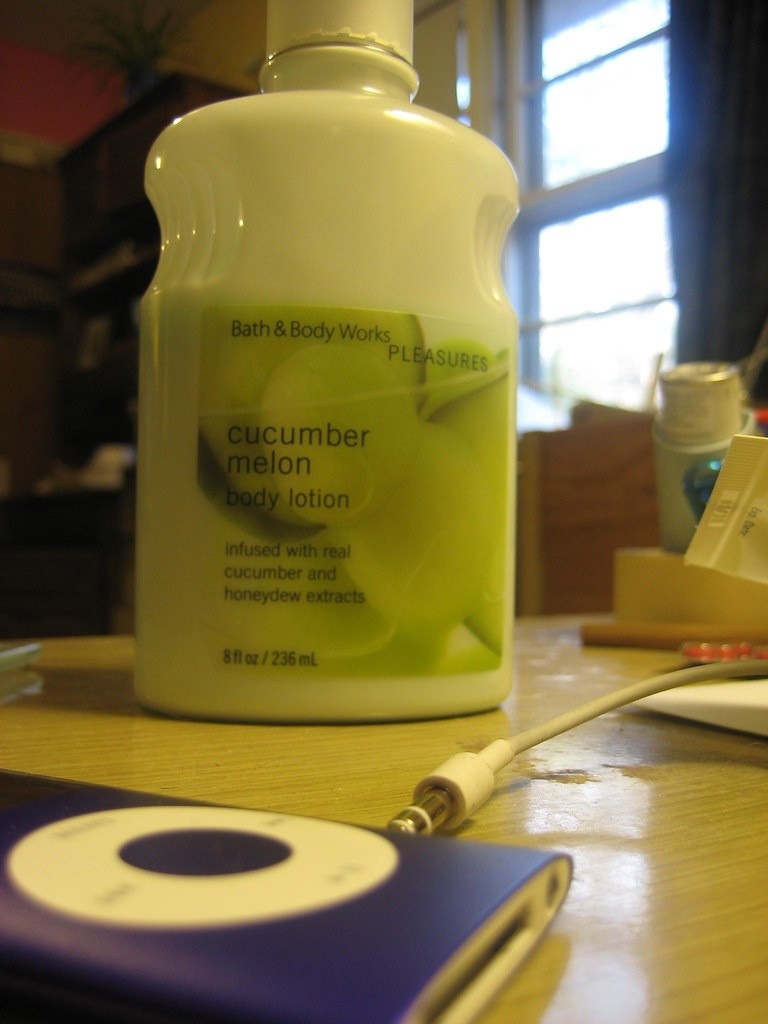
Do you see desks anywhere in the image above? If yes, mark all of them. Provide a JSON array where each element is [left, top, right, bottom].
[[1, 613, 768, 1024]]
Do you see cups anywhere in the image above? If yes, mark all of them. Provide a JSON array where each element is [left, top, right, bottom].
[[649, 407, 756, 552]]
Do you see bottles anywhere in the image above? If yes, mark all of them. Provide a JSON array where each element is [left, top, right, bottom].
[[136, 0, 522, 724]]
